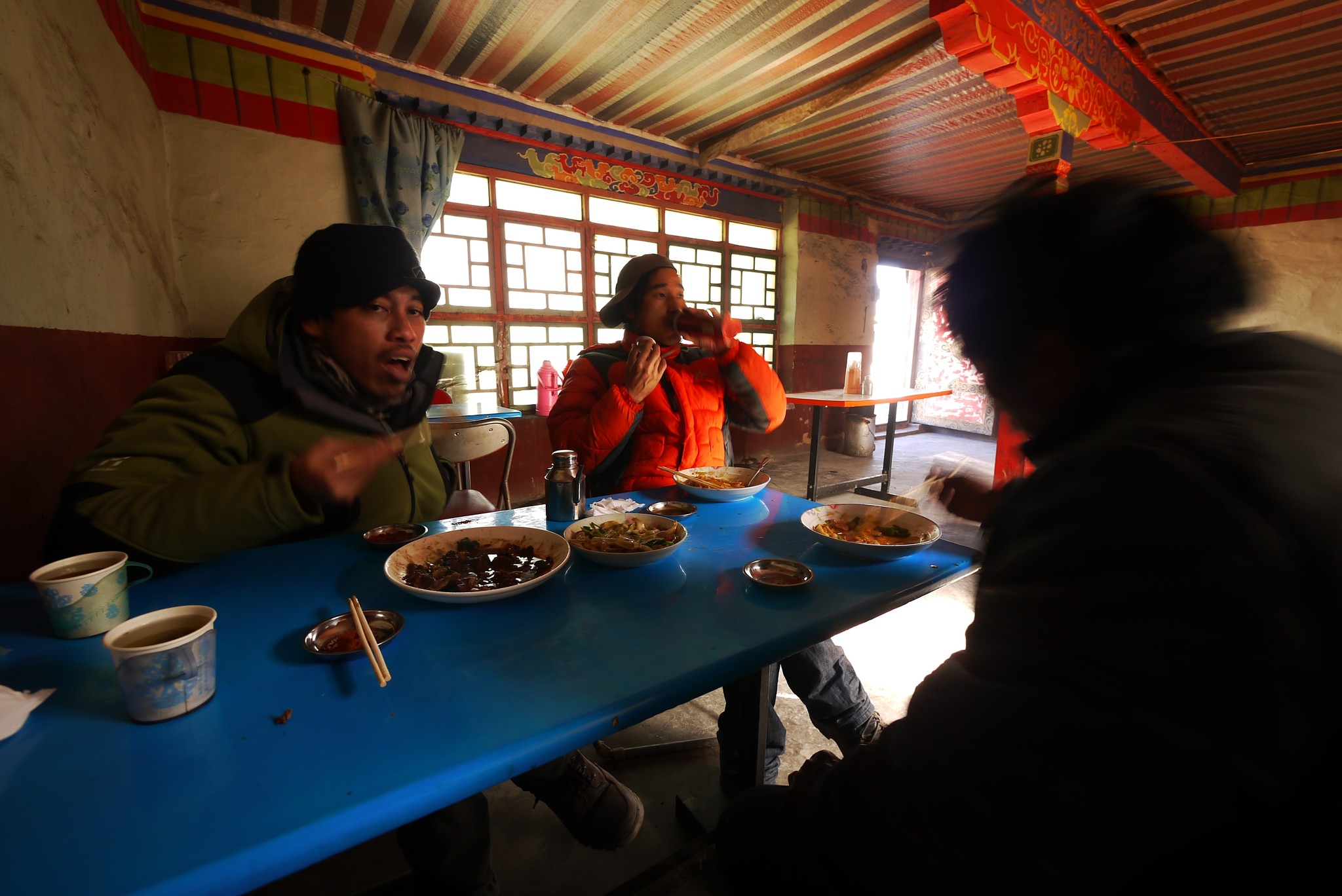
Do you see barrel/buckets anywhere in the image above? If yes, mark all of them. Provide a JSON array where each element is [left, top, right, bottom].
[[844, 404, 877, 457]]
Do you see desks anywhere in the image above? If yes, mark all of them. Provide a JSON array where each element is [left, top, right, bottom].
[[427, 404, 522, 490], [786, 387, 952, 508], [0, 479, 980, 896]]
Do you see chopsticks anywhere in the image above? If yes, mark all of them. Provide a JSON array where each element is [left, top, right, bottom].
[[349, 595, 391, 688], [800, 504, 942, 561], [874, 456, 970, 526], [657, 465, 726, 489]]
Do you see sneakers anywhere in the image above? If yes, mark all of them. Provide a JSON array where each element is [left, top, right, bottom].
[[809, 702, 888, 758], [531, 749, 644, 849]]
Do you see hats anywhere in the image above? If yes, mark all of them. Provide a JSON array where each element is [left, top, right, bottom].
[[600, 254, 678, 328], [288, 223, 442, 320]]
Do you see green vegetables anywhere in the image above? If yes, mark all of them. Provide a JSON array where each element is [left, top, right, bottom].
[[580, 522, 674, 548]]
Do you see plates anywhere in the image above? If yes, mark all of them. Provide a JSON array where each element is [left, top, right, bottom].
[[384, 526, 571, 603], [564, 513, 688, 566], [672, 466, 771, 502], [303, 610, 405, 660], [362, 522, 429, 549], [647, 500, 698, 521], [742, 559, 814, 587]]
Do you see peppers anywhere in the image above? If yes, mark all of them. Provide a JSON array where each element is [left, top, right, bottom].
[[884, 524, 911, 537]]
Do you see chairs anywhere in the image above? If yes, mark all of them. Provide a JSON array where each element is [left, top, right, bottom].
[[429, 417, 514, 511]]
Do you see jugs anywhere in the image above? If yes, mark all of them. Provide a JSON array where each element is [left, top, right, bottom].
[[562, 359, 574, 385], [544, 450, 586, 521], [535, 360, 564, 416], [861, 375, 874, 396]]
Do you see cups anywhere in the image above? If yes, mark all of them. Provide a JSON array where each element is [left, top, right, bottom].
[[29, 550, 128, 639], [103, 606, 217, 722]]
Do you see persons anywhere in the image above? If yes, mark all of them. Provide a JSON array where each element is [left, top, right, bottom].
[[54, 223, 645, 896], [549, 253, 887, 789], [684, 169, 1342, 895]]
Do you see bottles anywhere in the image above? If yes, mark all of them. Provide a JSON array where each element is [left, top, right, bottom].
[[843, 351, 862, 394], [674, 311, 742, 339]]
